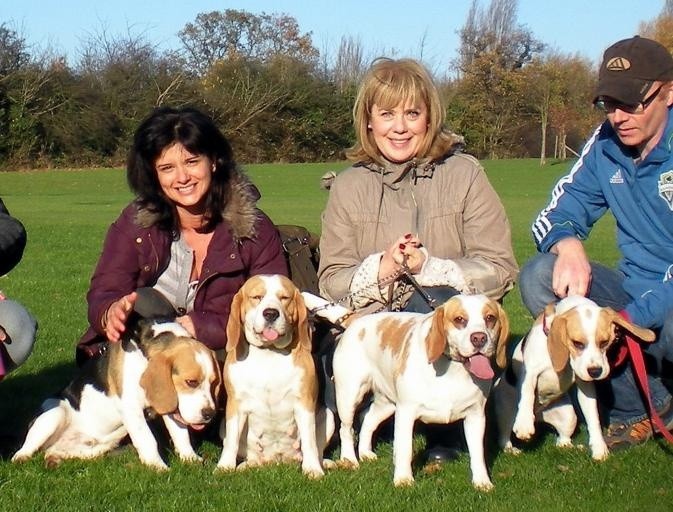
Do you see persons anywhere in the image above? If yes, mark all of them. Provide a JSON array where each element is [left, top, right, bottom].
[[62, 104, 294, 448], [314, 55, 520, 464], [0, 194, 41, 381], [513, 33, 673, 452]]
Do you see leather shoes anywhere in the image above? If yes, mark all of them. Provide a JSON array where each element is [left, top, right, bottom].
[[412, 443, 459, 465]]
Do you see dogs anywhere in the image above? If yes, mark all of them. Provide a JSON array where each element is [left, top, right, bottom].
[[301, 291, 511, 494], [10, 316, 223, 474], [211, 273, 339, 480], [492, 295, 657, 464]]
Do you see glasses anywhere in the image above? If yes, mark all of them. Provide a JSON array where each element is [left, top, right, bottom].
[[595, 79, 666, 114]]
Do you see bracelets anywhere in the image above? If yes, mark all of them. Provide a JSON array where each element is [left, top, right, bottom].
[[102, 303, 109, 331]]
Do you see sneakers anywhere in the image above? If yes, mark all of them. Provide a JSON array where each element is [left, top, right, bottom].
[[602, 397, 672, 452]]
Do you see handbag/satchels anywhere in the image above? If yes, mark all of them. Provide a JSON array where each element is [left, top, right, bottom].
[[275, 224, 322, 300]]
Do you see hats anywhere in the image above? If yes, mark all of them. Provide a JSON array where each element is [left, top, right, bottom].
[[590, 35, 672, 112]]
